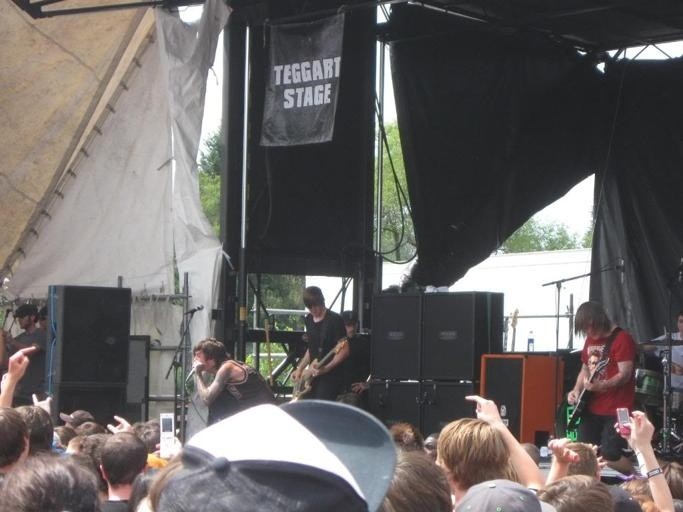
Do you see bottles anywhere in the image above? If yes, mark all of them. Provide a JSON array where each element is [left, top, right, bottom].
[[527, 330, 534, 352], [660, 427, 670, 454]]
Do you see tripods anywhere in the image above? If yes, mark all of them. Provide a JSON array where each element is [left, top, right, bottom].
[[653, 288, 683, 461]]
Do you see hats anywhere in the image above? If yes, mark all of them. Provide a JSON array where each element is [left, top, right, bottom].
[[157, 399, 397, 512], [340, 311, 358, 324], [13, 305, 38, 317], [60, 410, 95, 426], [609, 487, 643, 512], [454, 479, 557, 512]]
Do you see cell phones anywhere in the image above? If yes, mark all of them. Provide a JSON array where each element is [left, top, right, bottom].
[[159, 414, 175, 458], [617, 407, 630, 439]]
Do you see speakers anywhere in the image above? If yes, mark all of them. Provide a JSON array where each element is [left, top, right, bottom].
[[422, 292, 504, 434], [185, 370, 241, 447], [480, 353, 565, 456], [370, 293, 421, 434], [45, 285, 132, 429]]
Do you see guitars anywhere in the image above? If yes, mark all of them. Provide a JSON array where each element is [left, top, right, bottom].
[[568, 357, 610, 431], [292, 337, 347, 399]]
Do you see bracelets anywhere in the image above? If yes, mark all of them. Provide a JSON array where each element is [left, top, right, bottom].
[[644, 468, 664, 478]]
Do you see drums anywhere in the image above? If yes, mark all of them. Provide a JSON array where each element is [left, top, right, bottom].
[[634, 369, 663, 396], [667, 389, 683, 412]]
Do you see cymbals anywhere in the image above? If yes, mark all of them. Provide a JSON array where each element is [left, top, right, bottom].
[[640, 340, 683, 346]]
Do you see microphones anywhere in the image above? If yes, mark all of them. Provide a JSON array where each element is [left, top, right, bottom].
[[621, 258, 625, 284], [185, 304, 204, 314], [677, 256, 683, 282], [185, 366, 195, 383]]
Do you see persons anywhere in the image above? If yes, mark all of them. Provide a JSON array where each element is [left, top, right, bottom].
[[639, 313, 683, 390], [566, 301, 637, 461], [192, 338, 272, 417], [339, 310, 371, 399], [1, 345, 683, 512], [2, 303, 49, 408], [290, 287, 350, 400]]
